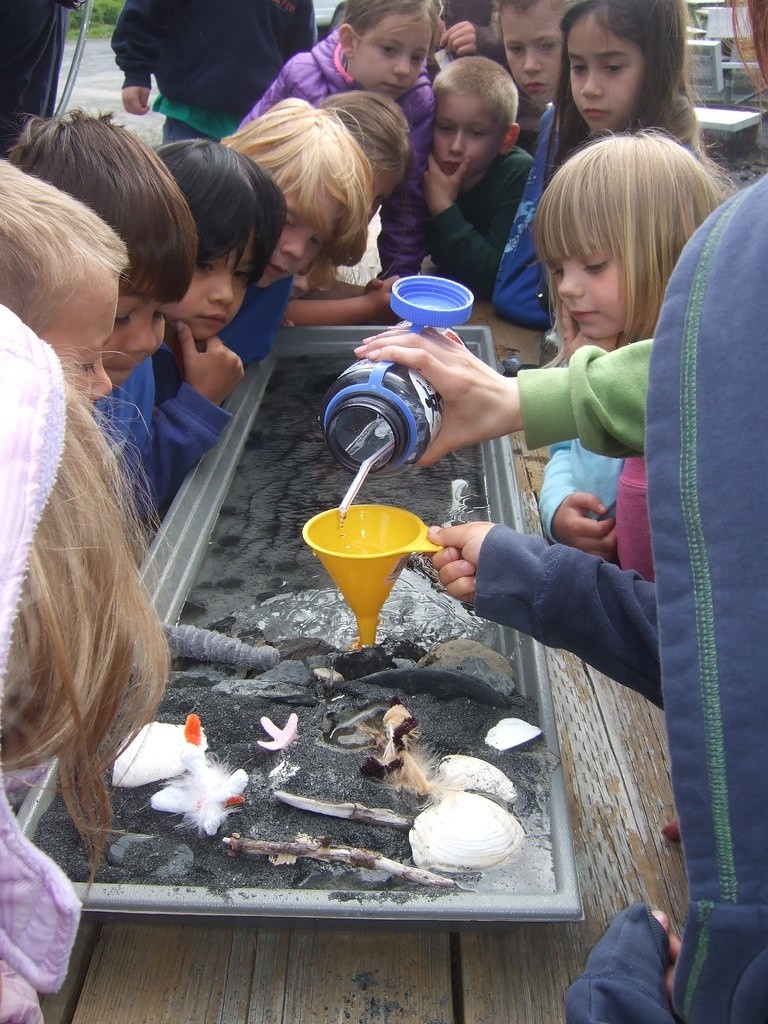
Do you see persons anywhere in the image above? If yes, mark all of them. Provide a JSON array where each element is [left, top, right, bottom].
[[0, 0, 768, 1024]]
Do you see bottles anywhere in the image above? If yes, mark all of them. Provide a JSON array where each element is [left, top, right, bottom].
[[320, 274, 474, 477]]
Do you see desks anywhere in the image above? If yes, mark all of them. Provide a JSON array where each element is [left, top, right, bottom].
[[41, 300, 693, 1022]]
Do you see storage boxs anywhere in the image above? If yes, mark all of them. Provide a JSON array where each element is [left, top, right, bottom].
[[695, 108, 761, 156]]
